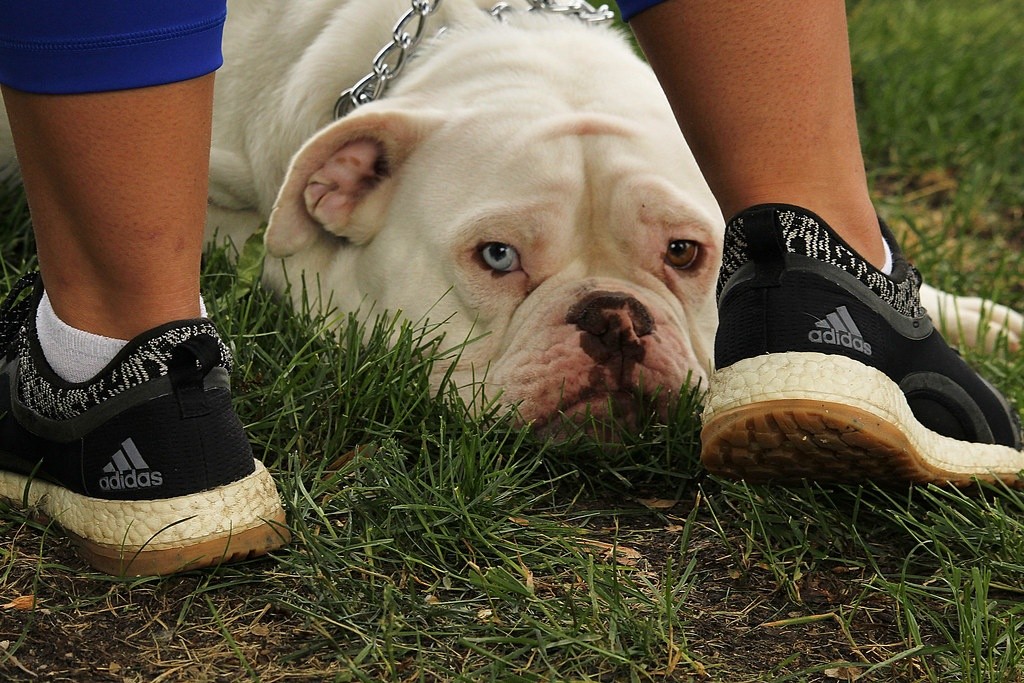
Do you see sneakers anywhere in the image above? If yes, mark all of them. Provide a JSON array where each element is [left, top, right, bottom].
[[699, 201, 1024, 486], [0, 271, 292, 581]]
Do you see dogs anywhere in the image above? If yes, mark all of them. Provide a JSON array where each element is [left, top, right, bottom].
[[1, 2, 1024, 461]]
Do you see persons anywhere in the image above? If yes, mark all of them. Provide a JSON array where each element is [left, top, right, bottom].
[[0, 0, 1024, 582]]
